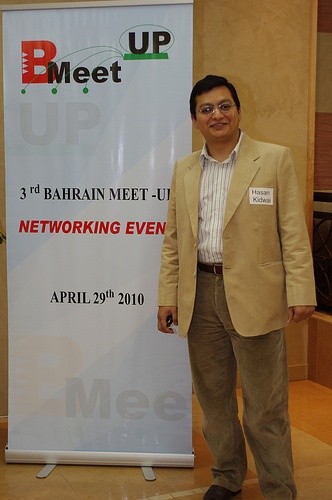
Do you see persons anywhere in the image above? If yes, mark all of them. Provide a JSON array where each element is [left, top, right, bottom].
[[157, 74, 317, 500]]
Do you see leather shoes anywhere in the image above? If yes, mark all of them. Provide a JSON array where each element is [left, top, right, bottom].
[[202, 485, 242, 500]]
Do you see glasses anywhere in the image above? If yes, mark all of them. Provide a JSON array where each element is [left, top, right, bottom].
[[196, 103, 236, 115]]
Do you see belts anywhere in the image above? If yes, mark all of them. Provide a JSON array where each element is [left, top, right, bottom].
[[197, 262, 223, 275]]
